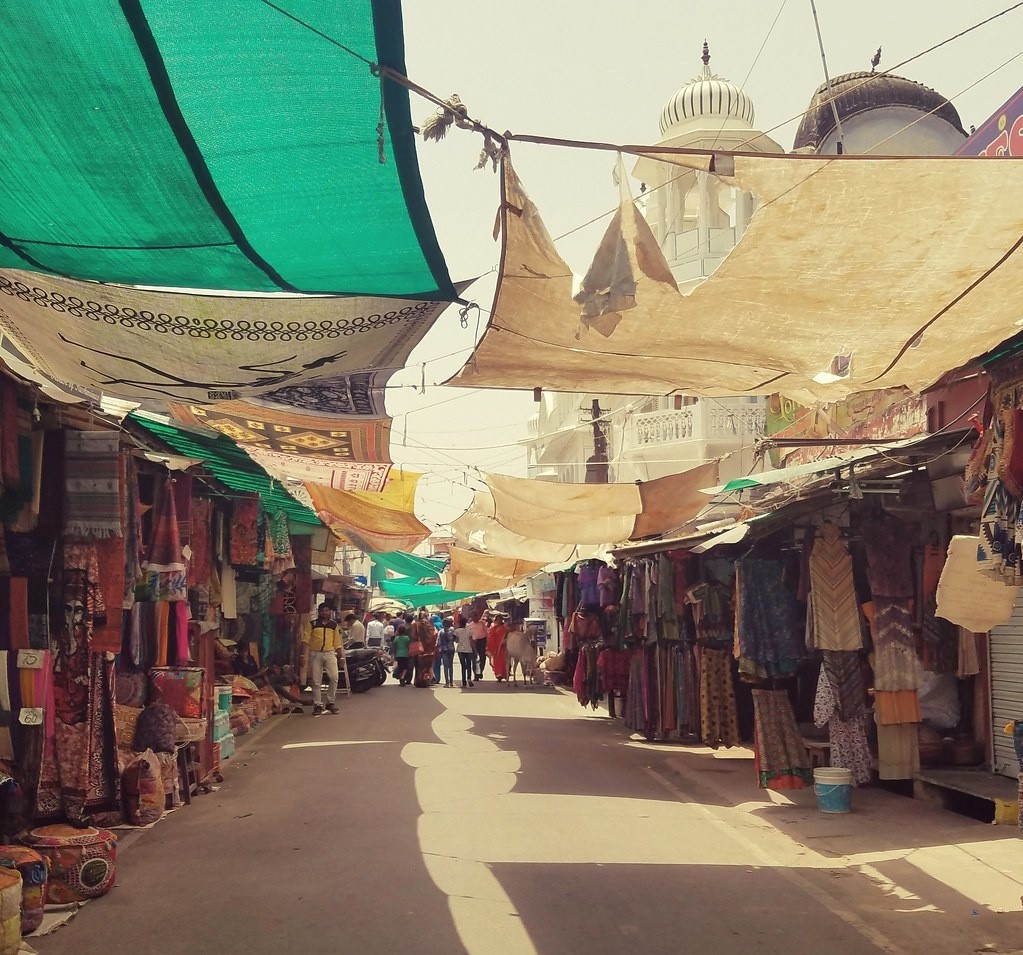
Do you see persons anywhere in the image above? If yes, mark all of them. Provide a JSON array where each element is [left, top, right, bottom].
[[299, 602, 345, 718], [231, 642, 313, 709], [344, 614, 365, 650], [366, 606, 510, 688]]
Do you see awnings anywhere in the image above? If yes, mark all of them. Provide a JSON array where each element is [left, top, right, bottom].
[[606, 426, 980, 559], [0, 307, 102, 404], [91, 393, 322, 525]]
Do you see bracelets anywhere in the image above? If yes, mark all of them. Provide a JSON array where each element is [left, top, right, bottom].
[[339, 657, 345, 660], [300, 655, 305, 658]]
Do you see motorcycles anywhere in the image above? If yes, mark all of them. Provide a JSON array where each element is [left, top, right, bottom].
[[339, 640, 392, 692]]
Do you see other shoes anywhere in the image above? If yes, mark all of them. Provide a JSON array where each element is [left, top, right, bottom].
[[468, 679, 474, 687], [314, 705, 321, 716], [479, 669, 483, 679], [291, 707, 304, 713], [282, 707, 289, 714], [325, 703, 339, 714], [449, 679, 453, 687], [400, 678, 406, 687], [473, 675, 478, 681], [443, 684, 449, 688], [462, 679, 466, 687]]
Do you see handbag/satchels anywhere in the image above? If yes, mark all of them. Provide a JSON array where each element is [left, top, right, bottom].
[[996, 406, 1023, 498], [964, 424, 993, 505], [408, 641, 424, 656]]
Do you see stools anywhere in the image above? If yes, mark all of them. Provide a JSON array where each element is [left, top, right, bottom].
[[810, 748, 829, 769], [0, 822, 121, 955]]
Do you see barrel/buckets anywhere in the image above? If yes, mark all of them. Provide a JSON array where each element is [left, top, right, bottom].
[[812, 768, 852, 814]]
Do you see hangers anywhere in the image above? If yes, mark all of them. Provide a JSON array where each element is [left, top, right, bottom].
[[758, 678, 781, 689], [681, 568, 731, 594], [738, 544, 761, 561], [872, 507, 896, 519], [804, 514, 855, 540], [912, 531, 948, 556], [61, 399, 122, 433]]
